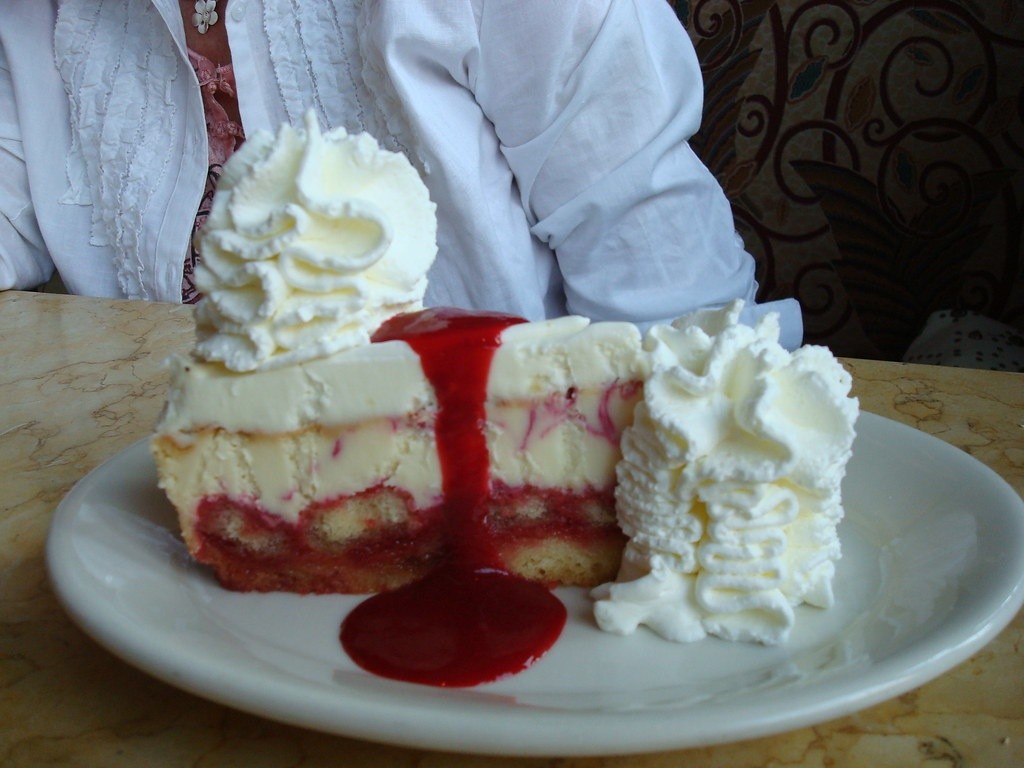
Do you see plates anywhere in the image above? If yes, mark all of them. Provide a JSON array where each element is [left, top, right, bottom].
[[46, 412, 1024, 758]]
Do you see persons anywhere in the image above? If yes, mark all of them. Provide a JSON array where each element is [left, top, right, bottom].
[[1, 0, 803, 354]]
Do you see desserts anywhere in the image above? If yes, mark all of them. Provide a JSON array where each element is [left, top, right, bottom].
[[147, 109, 862, 647]]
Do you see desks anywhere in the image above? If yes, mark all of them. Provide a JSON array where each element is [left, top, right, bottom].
[[0, 290, 1024, 768]]
